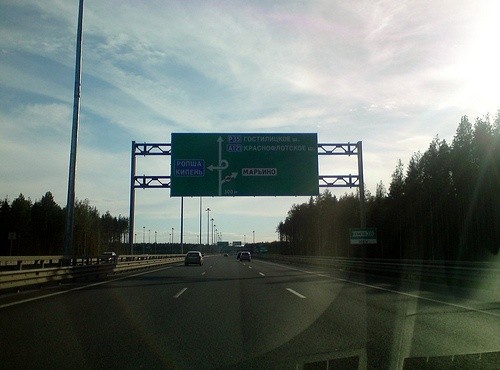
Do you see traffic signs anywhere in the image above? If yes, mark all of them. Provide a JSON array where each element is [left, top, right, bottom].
[[170, 132, 319, 198]]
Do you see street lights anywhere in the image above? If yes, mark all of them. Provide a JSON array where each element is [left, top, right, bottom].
[[143, 227, 145, 242], [205, 207, 211, 245], [214, 224, 222, 243], [211, 217, 214, 245], [253, 230, 255, 243], [172, 227, 174, 243], [169, 234, 171, 243], [155, 231, 157, 243], [148, 229, 150, 242]]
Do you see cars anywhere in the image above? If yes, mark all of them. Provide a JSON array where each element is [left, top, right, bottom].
[[237, 252, 241, 260], [239, 251, 251, 262], [183, 251, 205, 266], [224, 253, 228, 257], [100, 252, 117, 264], [58, 255, 77, 266]]
